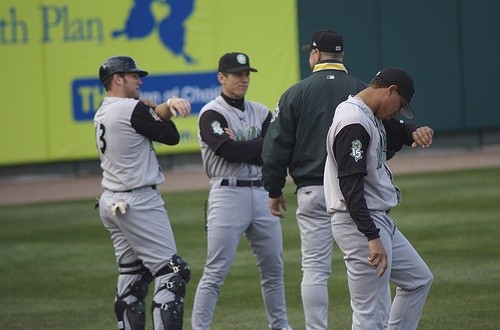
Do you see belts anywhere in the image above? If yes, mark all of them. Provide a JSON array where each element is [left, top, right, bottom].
[[114, 183, 156, 193], [221, 179, 263, 187]]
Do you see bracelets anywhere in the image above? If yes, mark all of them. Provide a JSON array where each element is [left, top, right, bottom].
[[167, 96, 176, 106]]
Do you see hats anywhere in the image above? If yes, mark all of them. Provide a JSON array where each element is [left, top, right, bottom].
[[218, 52, 257, 73], [376, 67, 415, 120], [301, 30, 344, 53]]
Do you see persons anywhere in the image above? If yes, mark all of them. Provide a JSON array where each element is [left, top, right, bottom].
[[323, 66, 433, 330], [94, 56, 191, 330], [261, 30, 406, 330], [191, 52, 293, 330]]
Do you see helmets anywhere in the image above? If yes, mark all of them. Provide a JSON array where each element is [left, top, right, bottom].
[[99, 56, 149, 82]]
